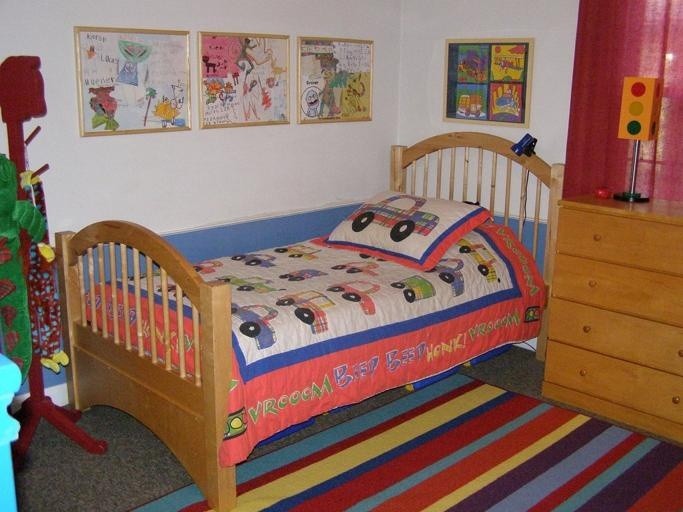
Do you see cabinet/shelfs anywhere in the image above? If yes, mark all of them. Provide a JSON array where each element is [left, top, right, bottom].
[[539, 195, 683, 446]]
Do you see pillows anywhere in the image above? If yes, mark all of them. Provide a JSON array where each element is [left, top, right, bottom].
[[322, 187, 492, 274]]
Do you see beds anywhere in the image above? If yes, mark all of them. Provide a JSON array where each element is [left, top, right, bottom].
[[53, 132, 566, 511]]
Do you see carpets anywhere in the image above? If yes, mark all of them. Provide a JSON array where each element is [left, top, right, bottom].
[[131, 370, 682, 512]]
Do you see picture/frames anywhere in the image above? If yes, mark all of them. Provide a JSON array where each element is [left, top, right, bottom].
[[74, 28, 192, 139], [440, 35, 535, 130], [297, 39, 371, 138], [197, 26, 293, 129]]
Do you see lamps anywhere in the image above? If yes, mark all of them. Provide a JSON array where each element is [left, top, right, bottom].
[[613, 73, 664, 204], [508, 132, 538, 158]]
